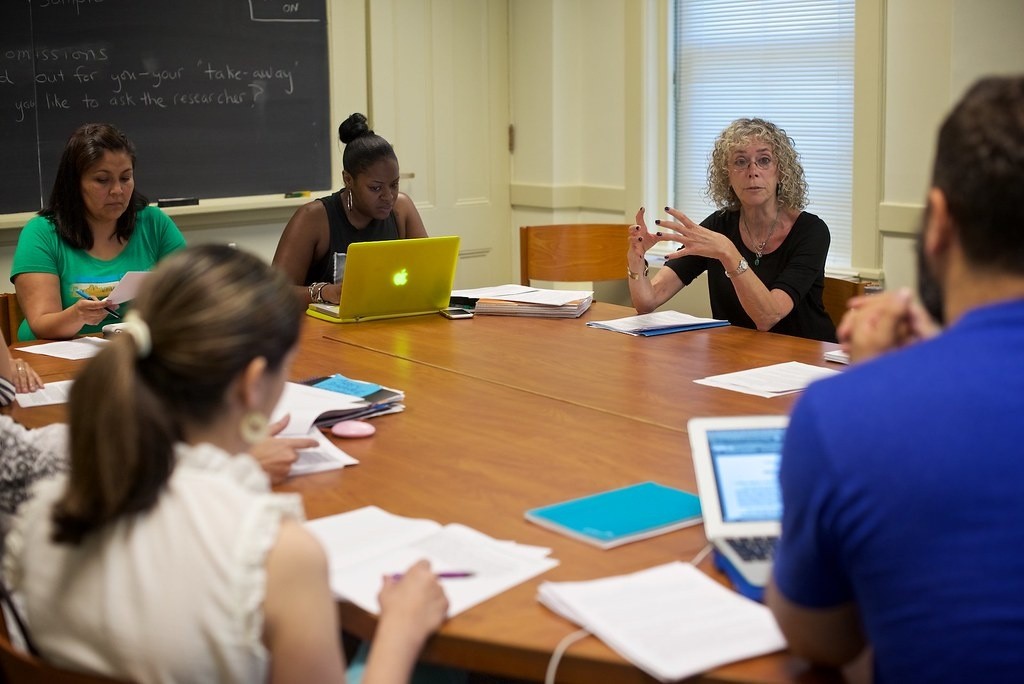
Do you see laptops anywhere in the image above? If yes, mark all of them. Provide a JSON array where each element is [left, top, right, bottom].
[[308, 235, 461, 321], [688, 415, 791, 586]]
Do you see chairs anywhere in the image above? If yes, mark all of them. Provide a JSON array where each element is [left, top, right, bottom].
[[520, 224, 629, 304]]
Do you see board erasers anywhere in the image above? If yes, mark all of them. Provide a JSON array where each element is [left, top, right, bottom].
[[158, 197, 199, 208]]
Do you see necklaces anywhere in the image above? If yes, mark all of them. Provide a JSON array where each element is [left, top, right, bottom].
[[741, 204, 780, 267]]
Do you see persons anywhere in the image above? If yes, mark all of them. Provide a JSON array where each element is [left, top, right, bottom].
[[0, 313, 319, 558], [9, 123, 187, 341], [0, 244, 449, 684], [624, 118, 838, 343], [766, 73, 1024, 684], [272, 113, 429, 310]]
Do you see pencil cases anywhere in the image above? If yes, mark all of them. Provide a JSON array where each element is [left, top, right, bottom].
[[102, 323, 126, 339]]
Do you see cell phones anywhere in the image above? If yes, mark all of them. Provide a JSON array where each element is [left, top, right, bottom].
[[438, 307, 472, 320]]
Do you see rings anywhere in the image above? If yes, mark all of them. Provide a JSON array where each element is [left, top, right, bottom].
[[17, 367, 25, 371]]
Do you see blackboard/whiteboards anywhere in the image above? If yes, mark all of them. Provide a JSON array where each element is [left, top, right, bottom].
[[0, 0, 335, 229]]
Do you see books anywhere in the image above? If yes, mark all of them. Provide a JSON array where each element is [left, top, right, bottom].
[[475, 293, 593, 318], [522, 480, 703, 551]]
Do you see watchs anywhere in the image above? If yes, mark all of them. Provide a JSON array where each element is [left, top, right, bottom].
[[725, 257, 748, 278]]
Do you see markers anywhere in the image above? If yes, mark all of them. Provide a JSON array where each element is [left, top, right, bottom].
[[285, 191, 311, 198]]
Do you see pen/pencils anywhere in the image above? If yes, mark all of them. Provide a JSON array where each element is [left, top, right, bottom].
[[75, 290, 122, 319], [393, 573, 471, 580]]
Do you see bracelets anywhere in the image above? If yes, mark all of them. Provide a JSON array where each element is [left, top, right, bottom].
[[308, 282, 331, 304], [627, 258, 649, 280]]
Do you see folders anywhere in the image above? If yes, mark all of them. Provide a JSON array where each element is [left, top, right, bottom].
[[302, 377, 400, 427]]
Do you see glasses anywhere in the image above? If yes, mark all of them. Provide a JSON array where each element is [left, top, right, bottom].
[[727, 156, 780, 173]]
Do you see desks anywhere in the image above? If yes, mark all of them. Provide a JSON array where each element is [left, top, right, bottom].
[[0, 298, 846, 684]]
